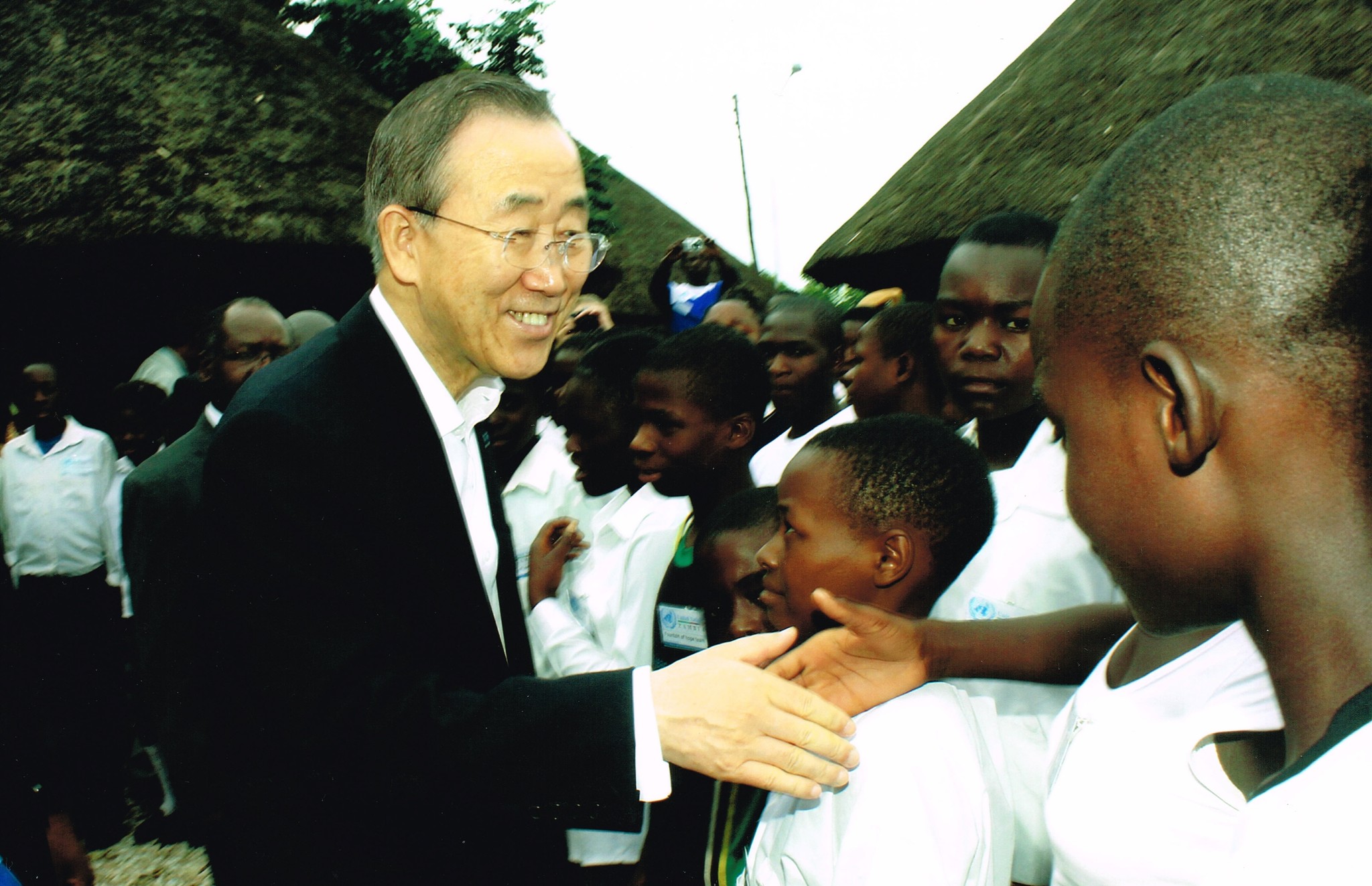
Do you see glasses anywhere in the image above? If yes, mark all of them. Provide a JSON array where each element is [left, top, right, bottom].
[[207, 338, 292, 365], [406, 206, 612, 274]]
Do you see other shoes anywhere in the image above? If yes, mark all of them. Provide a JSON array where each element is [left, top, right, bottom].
[[157, 810, 192, 845], [135, 809, 169, 844], [84, 824, 130, 850]]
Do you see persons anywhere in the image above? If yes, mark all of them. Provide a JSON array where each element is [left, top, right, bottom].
[[0, 72, 1369, 885]]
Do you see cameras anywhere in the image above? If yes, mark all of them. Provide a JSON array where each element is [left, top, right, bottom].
[[682, 236, 706, 255]]
